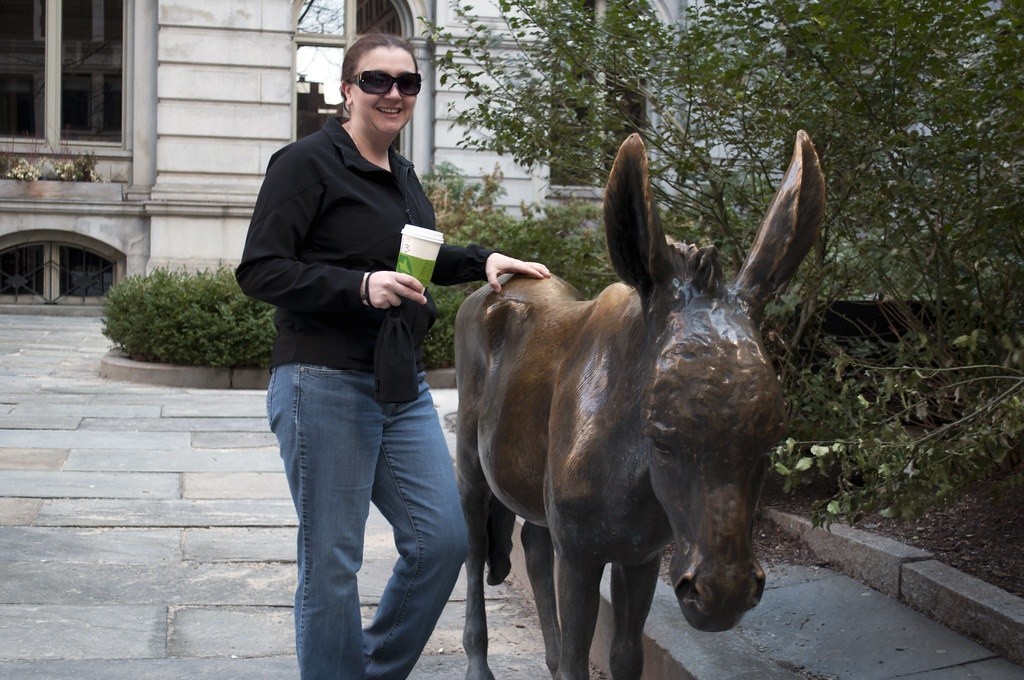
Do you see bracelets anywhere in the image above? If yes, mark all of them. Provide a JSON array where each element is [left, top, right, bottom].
[[362, 271, 375, 309]]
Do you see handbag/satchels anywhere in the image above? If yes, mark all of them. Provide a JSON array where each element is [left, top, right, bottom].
[[374, 307, 419, 404]]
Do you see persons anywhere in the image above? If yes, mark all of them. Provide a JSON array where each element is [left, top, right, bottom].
[[233, 35, 551, 680]]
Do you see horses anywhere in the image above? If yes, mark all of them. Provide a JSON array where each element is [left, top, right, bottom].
[[453, 127, 830, 680]]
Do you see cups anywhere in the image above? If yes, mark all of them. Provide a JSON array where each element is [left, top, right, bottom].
[[396, 224, 444, 293]]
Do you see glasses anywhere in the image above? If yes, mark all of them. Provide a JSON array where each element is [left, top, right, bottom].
[[349, 69, 422, 95]]
